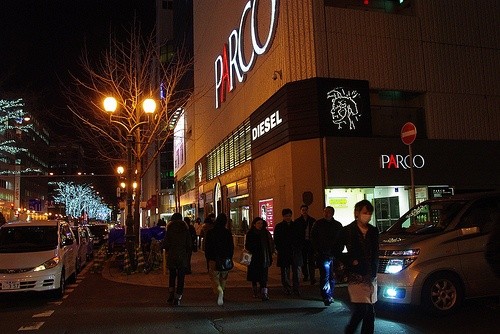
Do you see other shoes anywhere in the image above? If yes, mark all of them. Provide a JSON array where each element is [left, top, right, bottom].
[[303, 276, 308, 281], [285, 287, 291, 295], [324, 299, 330, 306], [294, 289, 301, 297], [175, 296, 183, 305], [311, 280, 317, 285], [330, 296, 334, 303], [167, 290, 175, 304], [218, 290, 224, 306]]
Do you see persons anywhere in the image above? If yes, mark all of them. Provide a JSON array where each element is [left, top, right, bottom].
[[184, 213, 216, 253], [332, 200, 379, 334], [157, 218, 166, 226], [274, 204, 344, 306], [245, 216, 276, 303], [205, 213, 234, 305], [161, 213, 192, 309]]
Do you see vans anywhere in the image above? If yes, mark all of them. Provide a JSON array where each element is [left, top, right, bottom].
[[342, 189, 499, 314], [0, 218, 81, 298]]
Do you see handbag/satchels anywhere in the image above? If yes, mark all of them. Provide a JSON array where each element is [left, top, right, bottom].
[[241, 251, 252, 265], [214, 254, 233, 271]]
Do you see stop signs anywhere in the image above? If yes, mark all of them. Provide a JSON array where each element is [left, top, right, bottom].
[[400, 122, 417, 145]]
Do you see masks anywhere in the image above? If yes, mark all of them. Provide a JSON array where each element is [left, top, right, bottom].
[[359, 213, 371, 224]]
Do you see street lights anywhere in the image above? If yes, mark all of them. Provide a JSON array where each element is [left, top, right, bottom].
[[102, 96, 157, 271]]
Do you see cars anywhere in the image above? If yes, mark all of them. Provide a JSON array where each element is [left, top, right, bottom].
[[70, 224, 109, 261]]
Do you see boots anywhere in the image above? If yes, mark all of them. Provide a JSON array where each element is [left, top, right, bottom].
[[252, 285, 258, 297], [261, 287, 269, 301]]
